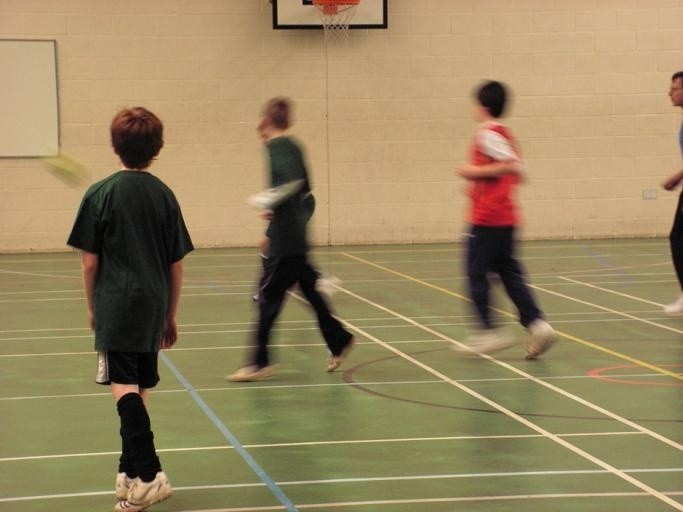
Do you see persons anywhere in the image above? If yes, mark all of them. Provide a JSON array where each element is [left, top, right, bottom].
[[660, 71, 682, 313], [223, 96, 355, 381], [453, 77, 556, 361], [250, 118, 341, 303], [66, 106, 194, 512]]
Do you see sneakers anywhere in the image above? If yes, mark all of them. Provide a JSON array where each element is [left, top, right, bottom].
[[325, 335, 356, 373], [114, 471, 174, 512], [226, 362, 273, 382], [523, 318, 558, 360], [660, 294, 682, 318]]
